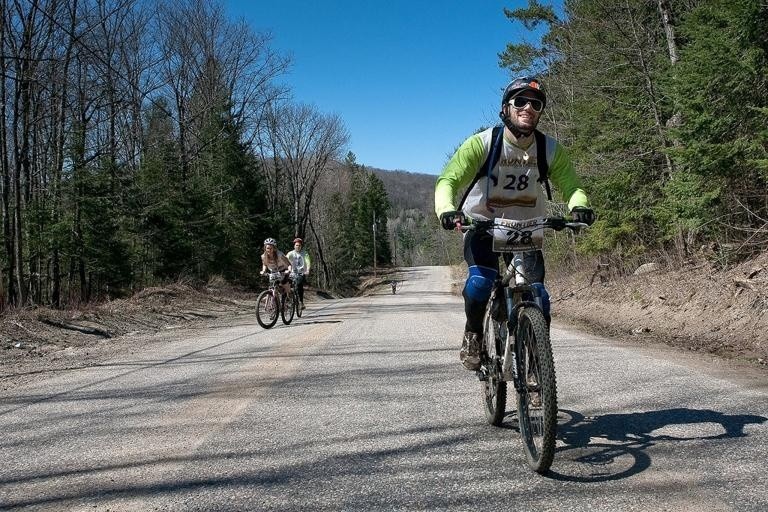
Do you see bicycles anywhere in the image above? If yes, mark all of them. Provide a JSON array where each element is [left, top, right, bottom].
[[451, 211, 589, 475], [254, 274, 303, 329]]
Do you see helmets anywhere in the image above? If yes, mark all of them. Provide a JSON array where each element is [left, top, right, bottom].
[[293, 238, 303, 244], [502, 75, 547, 112], [263, 237, 277, 249]]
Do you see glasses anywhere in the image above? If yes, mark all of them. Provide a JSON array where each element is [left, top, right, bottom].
[[508, 95, 545, 113]]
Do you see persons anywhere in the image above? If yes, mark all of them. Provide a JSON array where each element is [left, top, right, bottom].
[[434, 76, 596, 409], [260, 238, 312, 320]]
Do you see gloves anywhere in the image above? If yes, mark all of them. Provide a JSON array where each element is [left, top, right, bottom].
[[569, 205, 596, 235], [437, 208, 471, 237]]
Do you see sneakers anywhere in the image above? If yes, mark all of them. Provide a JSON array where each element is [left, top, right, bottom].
[[528, 373, 541, 404], [459, 318, 484, 372]]
